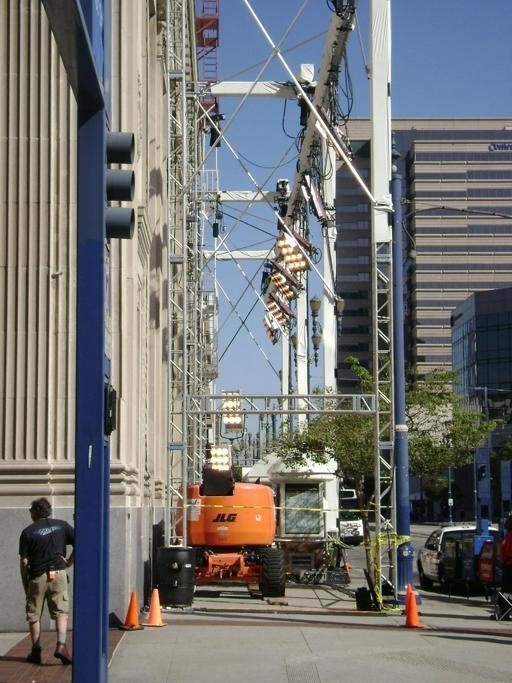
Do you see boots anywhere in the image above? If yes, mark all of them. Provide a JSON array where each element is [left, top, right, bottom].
[[27, 647, 41, 663], [54, 641, 73, 664]]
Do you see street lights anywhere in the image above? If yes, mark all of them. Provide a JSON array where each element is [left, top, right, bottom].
[[289, 297, 322, 426]]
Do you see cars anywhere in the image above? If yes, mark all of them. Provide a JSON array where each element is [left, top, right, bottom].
[[418, 522, 497, 593], [339, 489, 365, 548]]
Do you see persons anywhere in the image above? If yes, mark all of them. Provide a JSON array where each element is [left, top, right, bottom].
[[498, 515, 512, 591], [19, 497, 75, 665]]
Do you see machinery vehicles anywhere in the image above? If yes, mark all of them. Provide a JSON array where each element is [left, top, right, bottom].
[[155, 468, 285, 597]]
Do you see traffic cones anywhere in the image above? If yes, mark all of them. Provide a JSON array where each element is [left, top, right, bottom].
[[119, 591, 143, 630], [141, 588, 167, 626], [401, 584, 426, 630]]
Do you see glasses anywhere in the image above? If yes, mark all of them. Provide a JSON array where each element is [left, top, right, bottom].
[[29, 508, 33, 512]]
